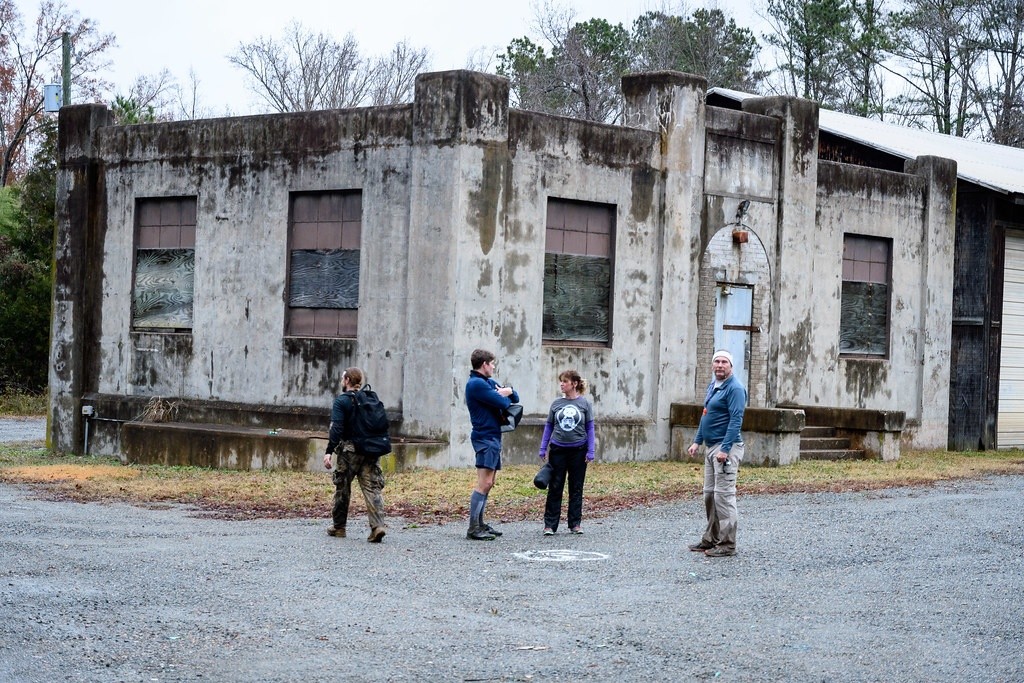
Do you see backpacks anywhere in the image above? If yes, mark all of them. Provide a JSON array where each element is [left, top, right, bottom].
[[339, 383, 392, 457]]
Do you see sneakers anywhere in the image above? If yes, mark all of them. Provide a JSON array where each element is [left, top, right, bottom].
[[568, 525, 583, 534], [367, 526, 386, 544], [327, 524, 346, 537], [688, 542, 713, 551], [479, 523, 502, 535], [705, 547, 736, 556], [466, 528, 496, 540], [543, 527, 554, 535]]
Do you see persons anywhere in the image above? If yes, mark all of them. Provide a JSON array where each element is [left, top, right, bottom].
[[323, 366, 386, 542], [687, 349, 747, 557], [465, 349, 519, 540], [539, 370, 595, 535]]
